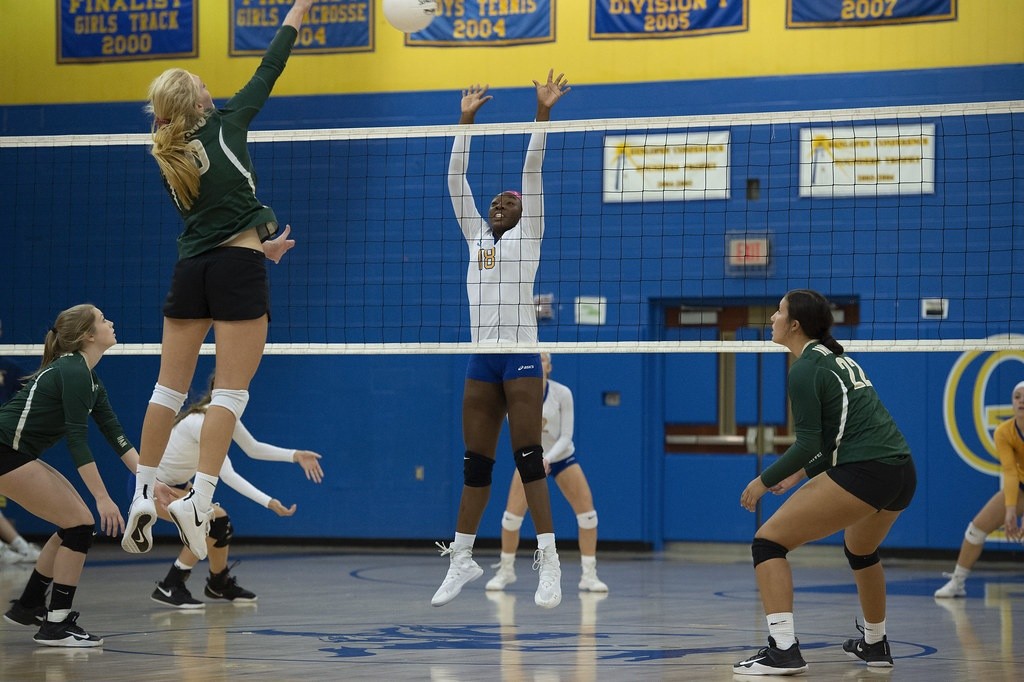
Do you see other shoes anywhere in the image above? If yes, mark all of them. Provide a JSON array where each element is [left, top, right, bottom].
[[934, 575, 967, 598], [934, 598, 967, 611]]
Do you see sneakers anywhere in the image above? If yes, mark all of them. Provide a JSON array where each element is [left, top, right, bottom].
[[120, 484, 158, 554], [167, 487, 220, 560], [841, 618, 895, 667], [578, 564, 608, 592], [150, 581, 206, 609], [431, 541, 484, 607], [485, 556, 517, 590], [0, 542, 43, 565], [578, 592, 609, 613], [32, 611, 104, 647], [2, 590, 51, 630], [532, 542, 562, 609], [201, 560, 257, 603], [732, 635, 809, 675], [486, 591, 516, 618]]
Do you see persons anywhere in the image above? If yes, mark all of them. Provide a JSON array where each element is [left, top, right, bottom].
[[122, 1, 314, 561], [485, 353, 608, 592], [934, 381, 1024, 598], [149, 375, 324, 610], [731, 289, 916, 674], [0, 357, 40, 564], [430, 68, 570, 609], [0, 301, 179, 648]]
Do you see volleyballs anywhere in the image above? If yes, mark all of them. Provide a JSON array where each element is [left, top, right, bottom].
[[382, 0, 438, 34]]
